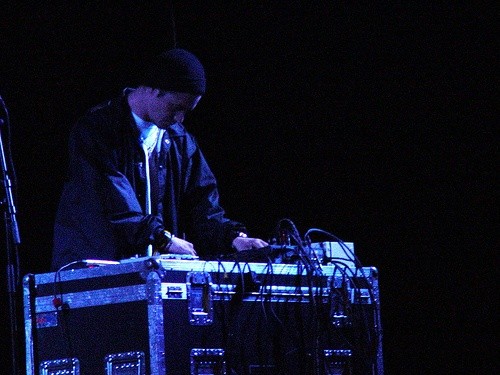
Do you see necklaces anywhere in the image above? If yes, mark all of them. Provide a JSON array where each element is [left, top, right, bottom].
[[139, 129, 159, 153]]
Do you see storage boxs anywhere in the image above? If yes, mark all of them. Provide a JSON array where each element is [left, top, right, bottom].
[[22, 258, 384, 375]]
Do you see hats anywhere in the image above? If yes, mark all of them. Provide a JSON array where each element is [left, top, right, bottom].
[[138, 48, 205, 95]]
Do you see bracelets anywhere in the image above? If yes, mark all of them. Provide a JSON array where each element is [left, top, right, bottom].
[[156, 231, 171, 251], [230, 232, 248, 247]]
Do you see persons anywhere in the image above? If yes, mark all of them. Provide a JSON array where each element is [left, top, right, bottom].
[[49, 48, 272, 278]]
